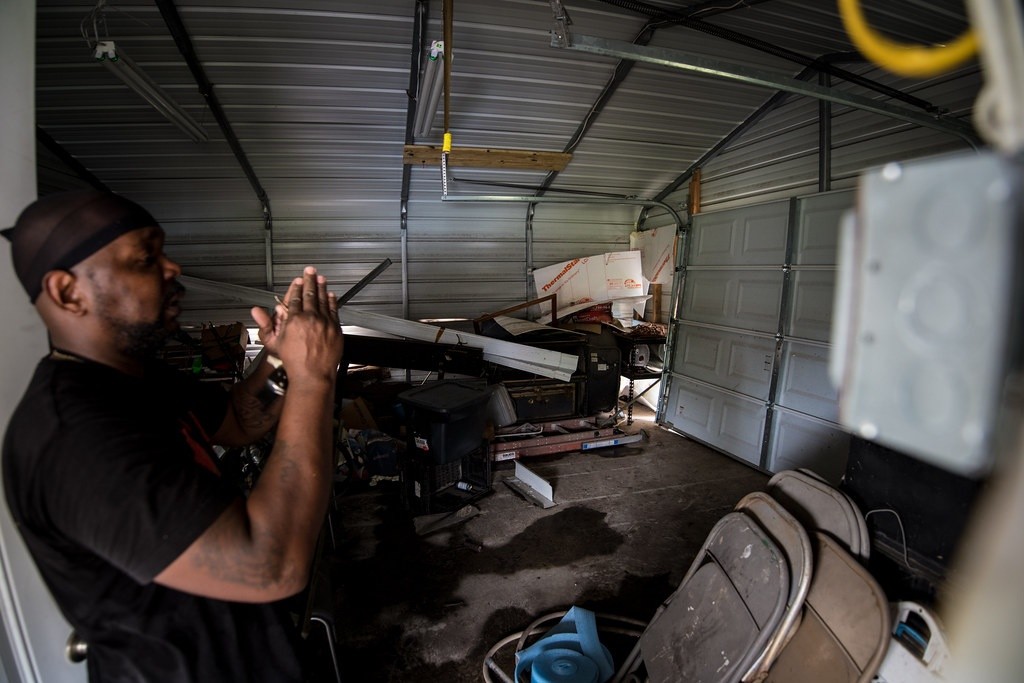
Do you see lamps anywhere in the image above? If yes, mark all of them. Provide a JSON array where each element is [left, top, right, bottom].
[[79, 0, 211, 145], [412, 0, 454, 138]]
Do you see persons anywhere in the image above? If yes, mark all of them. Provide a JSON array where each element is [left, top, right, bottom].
[[1, 182, 343, 682]]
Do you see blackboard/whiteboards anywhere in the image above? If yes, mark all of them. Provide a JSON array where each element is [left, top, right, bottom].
[[825, 150, 1021, 477]]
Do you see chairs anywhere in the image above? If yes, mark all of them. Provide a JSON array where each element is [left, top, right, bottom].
[[616, 467, 890, 683]]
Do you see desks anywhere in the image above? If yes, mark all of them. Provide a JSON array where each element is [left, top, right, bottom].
[[616, 333, 666, 426]]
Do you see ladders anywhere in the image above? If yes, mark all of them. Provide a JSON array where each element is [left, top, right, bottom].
[[493, 416, 650, 462]]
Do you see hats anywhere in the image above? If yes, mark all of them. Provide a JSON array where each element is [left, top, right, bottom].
[[0, 178, 160, 304]]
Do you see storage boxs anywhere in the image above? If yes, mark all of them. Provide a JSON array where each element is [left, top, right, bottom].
[[532, 251, 654, 324]]
